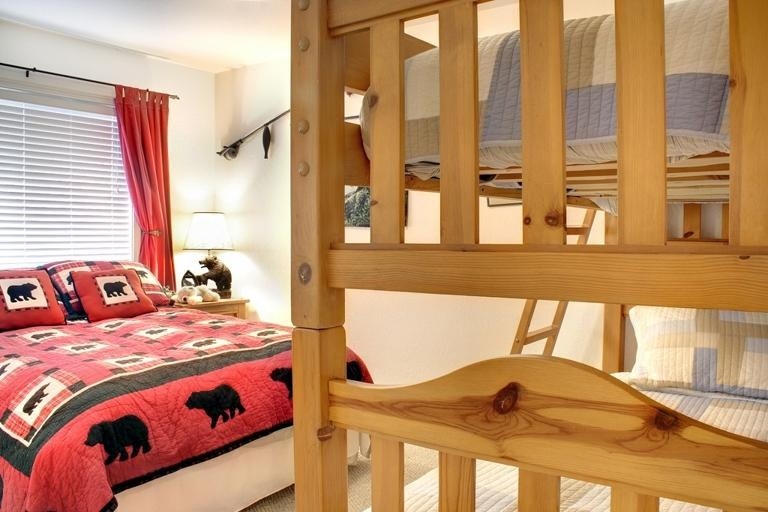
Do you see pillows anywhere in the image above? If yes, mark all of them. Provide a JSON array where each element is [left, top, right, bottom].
[[0, 259, 171, 334], [625, 304, 768, 400]]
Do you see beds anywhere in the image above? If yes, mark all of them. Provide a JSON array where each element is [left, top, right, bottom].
[[0, 303, 375, 512], [291, 2, 768, 511]]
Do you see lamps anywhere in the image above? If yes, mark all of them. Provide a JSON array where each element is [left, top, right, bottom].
[[182, 212, 235, 299]]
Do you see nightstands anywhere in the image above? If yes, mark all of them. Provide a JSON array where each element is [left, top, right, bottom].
[[174, 297, 250, 320]]
